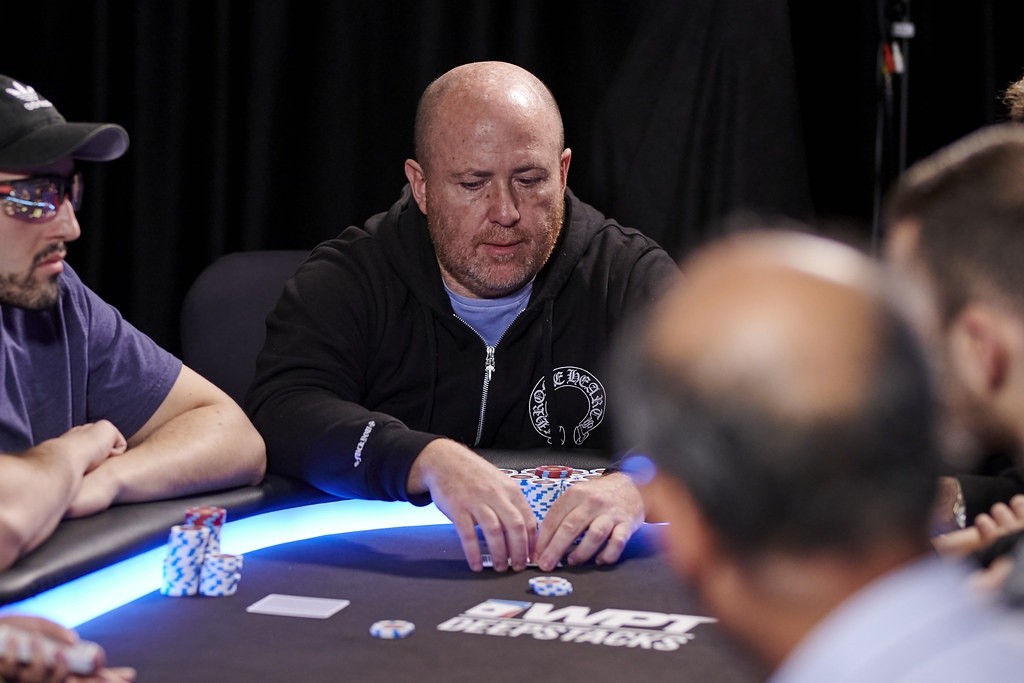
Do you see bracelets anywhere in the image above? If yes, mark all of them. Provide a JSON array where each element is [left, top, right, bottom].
[[952, 479, 966, 530]]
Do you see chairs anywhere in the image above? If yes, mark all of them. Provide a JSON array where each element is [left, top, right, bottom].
[[180, 248, 322, 413]]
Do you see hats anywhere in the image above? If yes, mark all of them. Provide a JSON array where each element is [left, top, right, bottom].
[[0, 74, 130, 171]]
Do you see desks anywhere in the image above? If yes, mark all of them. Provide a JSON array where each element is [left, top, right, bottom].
[[0, 443, 776, 683]]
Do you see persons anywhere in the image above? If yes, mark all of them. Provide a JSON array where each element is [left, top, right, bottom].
[[0, 72, 266, 572], [878, 80, 1024, 615], [609, 226, 1024, 683], [245, 62, 686, 577]]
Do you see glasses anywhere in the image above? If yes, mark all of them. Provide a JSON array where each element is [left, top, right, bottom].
[[0, 172, 83, 224]]
